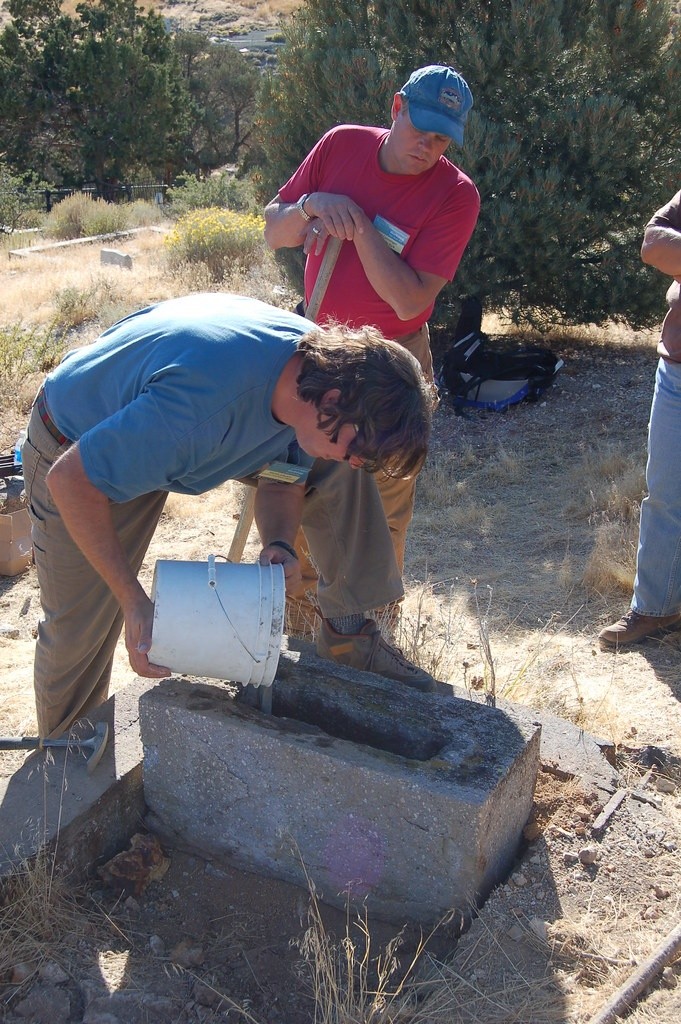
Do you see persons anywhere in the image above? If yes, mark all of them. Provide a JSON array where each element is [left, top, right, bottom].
[[598, 188, 681, 647], [20, 292, 455, 739], [264, 64, 481, 644]]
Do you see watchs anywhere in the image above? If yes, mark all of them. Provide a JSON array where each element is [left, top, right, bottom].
[[296, 192, 316, 223]]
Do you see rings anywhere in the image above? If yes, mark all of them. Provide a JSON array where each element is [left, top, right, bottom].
[[312, 227, 320, 234]]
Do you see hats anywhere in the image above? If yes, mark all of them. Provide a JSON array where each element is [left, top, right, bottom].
[[400, 65, 473, 146]]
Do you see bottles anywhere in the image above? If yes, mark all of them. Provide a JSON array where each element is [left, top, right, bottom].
[[14, 430, 27, 465]]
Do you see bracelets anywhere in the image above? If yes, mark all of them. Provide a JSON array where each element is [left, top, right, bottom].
[[268, 540, 298, 560]]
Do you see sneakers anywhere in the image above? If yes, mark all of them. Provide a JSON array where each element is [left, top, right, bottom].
[[317, 618, 436, 692], [599, 609, 681, 647]]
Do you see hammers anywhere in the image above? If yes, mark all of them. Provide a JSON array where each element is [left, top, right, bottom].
[[0, 721, 109, 772]]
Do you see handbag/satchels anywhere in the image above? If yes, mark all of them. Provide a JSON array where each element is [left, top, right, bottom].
[[439, 328, 564, 419]]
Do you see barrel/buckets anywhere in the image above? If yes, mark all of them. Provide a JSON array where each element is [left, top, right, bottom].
[[144, 555, 288, 688]]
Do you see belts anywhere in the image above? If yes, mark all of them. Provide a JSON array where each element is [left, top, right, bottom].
[[38, 386, 73, 451]]
